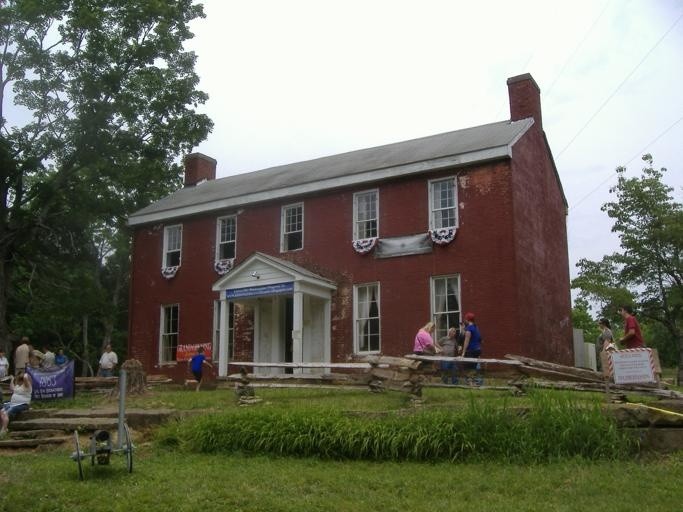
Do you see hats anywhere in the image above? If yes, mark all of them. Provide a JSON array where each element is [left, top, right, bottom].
[[466, 312, 474, 320]]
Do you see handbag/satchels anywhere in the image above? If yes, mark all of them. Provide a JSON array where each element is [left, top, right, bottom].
[[424, 349, 434, 356]]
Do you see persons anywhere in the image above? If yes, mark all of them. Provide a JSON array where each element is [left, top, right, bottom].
[[0, 335, 117, 437], [438, 327, 464, 387], [619, 305, 644, 350], [459, 312, 483, 386], [184, 346, 213, 394], [412, 322, 443, 372], [595, 318, 617, 354], [456, 320, 470, 385]]
